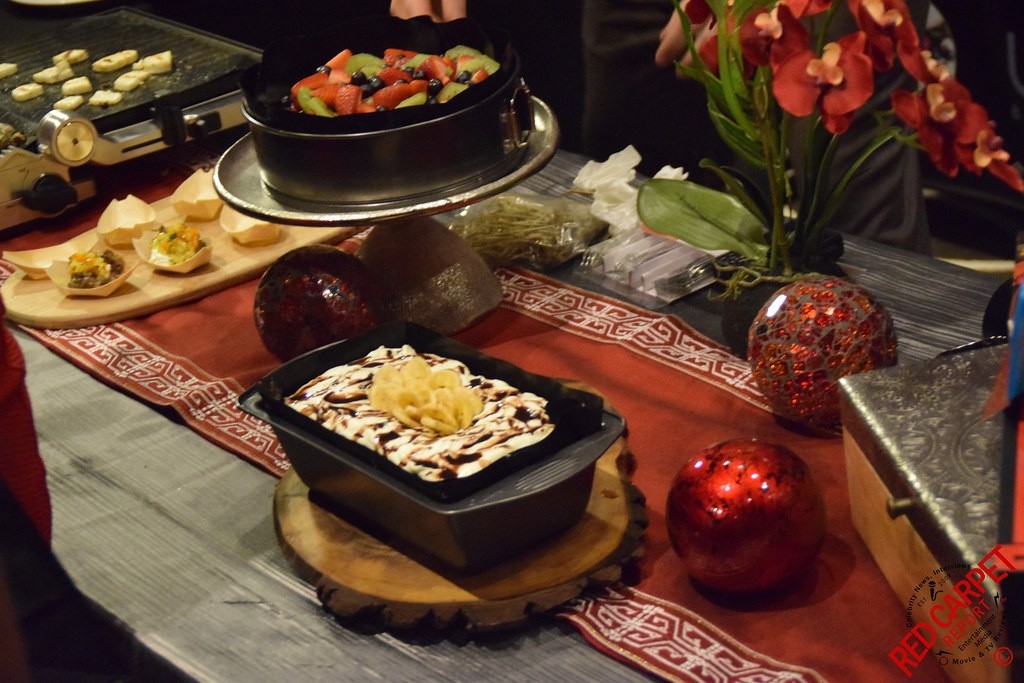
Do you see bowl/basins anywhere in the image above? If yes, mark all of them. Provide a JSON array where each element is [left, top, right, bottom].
[[240, 50, 537, 203], [235, 322, 626, 578]]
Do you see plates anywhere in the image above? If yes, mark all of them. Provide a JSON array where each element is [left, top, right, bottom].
[[0, 195, 371, 330]]
